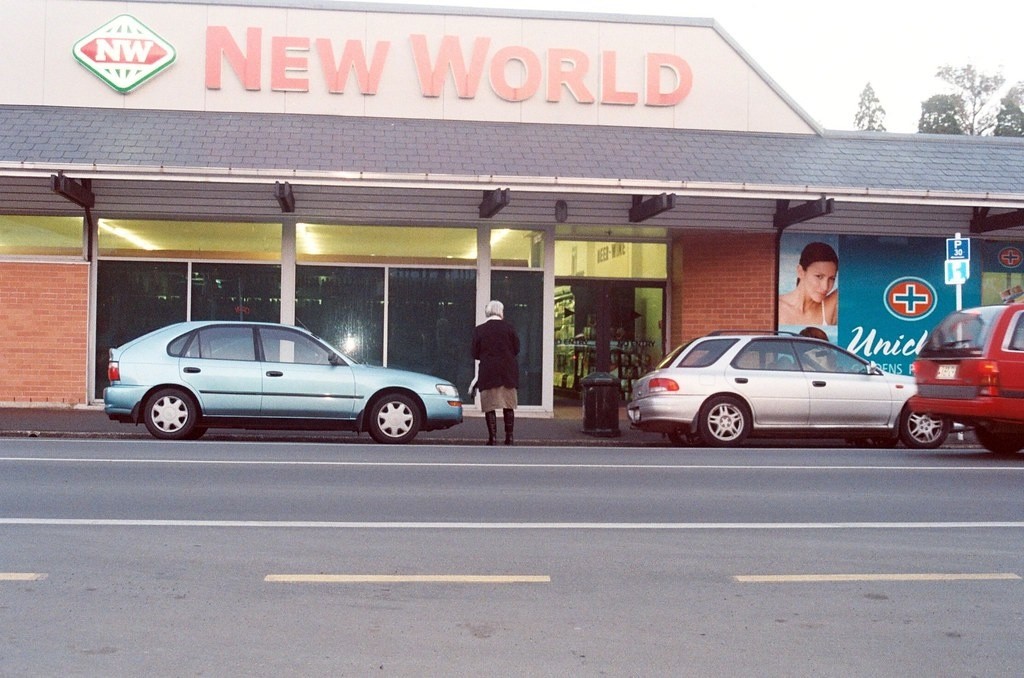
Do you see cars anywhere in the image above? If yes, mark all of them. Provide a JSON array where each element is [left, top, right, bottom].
[[625, 328, 975, 451], [102, 319, 465, 444]]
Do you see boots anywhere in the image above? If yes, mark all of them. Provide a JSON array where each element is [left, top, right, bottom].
[[503, 408, 514, 445], [486, 410, 497, 445]]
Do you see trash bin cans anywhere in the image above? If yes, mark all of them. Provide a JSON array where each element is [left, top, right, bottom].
[[580, 372, 621, 437]]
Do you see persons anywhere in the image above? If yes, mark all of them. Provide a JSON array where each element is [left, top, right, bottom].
[[470, 300, 521, 445], [777, 241, 838, 344], [795, 327, 843, 372]]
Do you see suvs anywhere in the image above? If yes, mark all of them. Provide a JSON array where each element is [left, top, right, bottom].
[[908, 303, 1024, 454]]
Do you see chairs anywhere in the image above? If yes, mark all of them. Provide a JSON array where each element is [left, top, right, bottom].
[[776, 357, 796, 371]]
